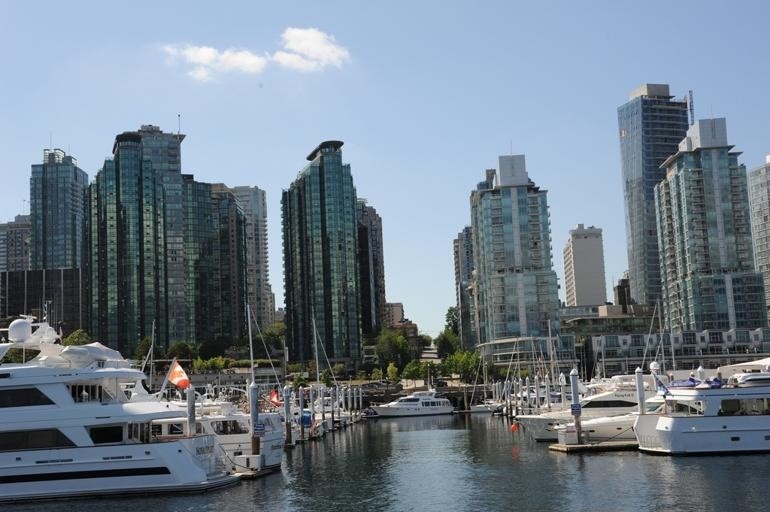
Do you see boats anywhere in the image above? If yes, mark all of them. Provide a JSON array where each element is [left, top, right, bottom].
[[368, 364, 455, 418]]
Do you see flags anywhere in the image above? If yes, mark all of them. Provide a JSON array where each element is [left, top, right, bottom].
[[168, 359, 190, 389], [270, 389, 281, 406]]
